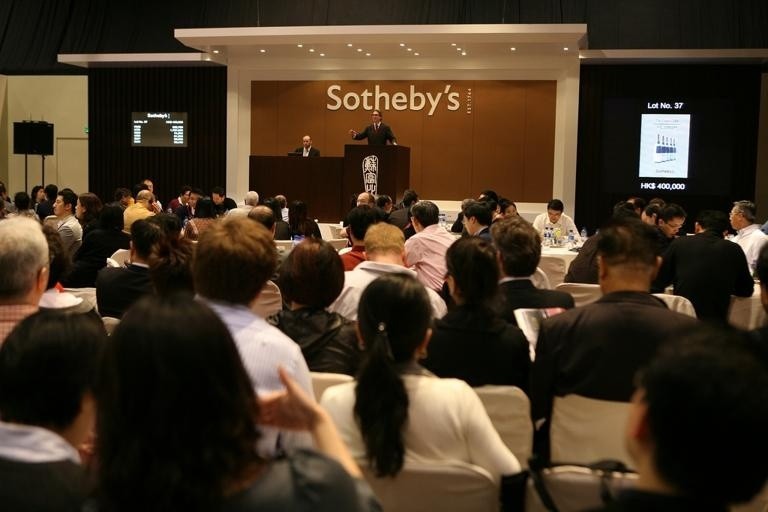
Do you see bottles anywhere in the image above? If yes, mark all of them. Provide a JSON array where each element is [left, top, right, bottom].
[[540, 226, 600, 248], [438, 213, 447, 229], [292, 234, 304, 246]]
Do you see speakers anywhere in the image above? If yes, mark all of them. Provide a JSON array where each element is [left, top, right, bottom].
[[14, 121, 54, 155]]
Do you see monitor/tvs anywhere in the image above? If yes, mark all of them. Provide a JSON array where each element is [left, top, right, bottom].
[[129, 109, 190, 149], [288, 152, 302, 156]]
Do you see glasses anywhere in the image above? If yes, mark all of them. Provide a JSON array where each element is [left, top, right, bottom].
[[461, 218, 472, 225], [410, 216, 417, 221]]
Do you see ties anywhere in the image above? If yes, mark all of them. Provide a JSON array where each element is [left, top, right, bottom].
[[376, 124, 378, 133]]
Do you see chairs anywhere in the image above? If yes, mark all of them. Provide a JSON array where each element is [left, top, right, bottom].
[[0, 219, 767, 512]]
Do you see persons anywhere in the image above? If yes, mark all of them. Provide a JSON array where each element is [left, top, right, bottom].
[[294, 135, 320, 158], [347, 110, 398, 146]]
